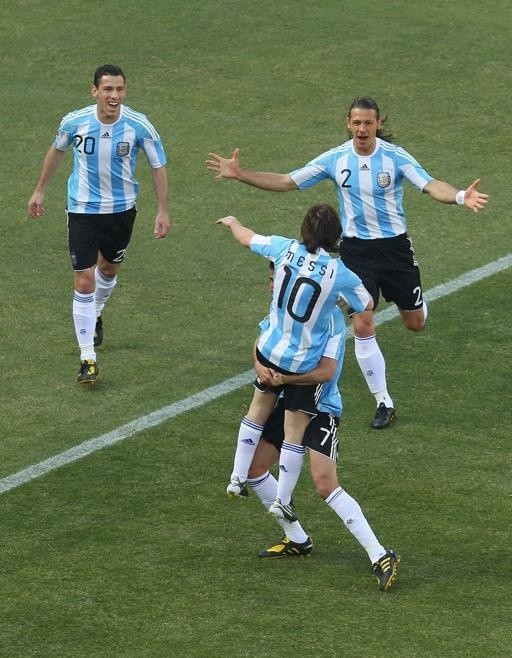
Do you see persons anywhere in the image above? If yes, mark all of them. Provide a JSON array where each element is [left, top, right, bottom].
[[28, 64, 170, 383], [214, 205, 375, 523], [204, 98, 489, 429], [246, 264, 402, 591]]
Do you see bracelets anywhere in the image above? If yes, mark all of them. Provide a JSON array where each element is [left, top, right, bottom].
[[454, 189, 466, 206]]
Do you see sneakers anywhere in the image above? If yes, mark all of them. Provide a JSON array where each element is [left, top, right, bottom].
[[227, 477, 249, 499], [259, 536, 314, 558], [269, 499, 297, 523], [93, 316, 103, 345], [372, 403, 396, 428], [370, 548, 402, 592], [79, 359, 99, 383]]
